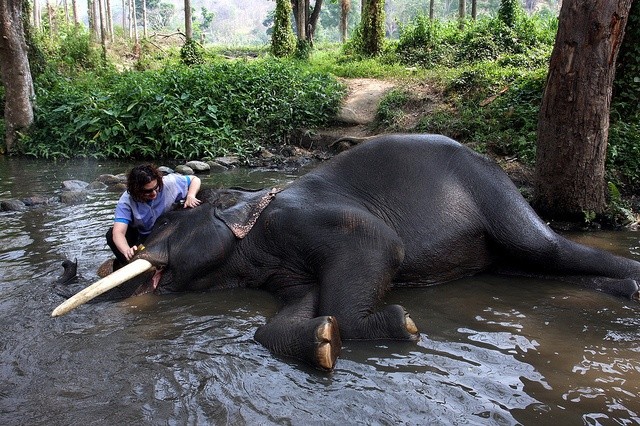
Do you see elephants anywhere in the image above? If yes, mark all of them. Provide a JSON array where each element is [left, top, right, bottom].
[[49, 131, 639, 374]]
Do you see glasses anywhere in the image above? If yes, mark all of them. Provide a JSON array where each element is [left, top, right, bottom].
[[144, 184, 158, 194]]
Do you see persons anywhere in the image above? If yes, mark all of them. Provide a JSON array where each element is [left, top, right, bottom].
[[106, 163, 203, 288]]
